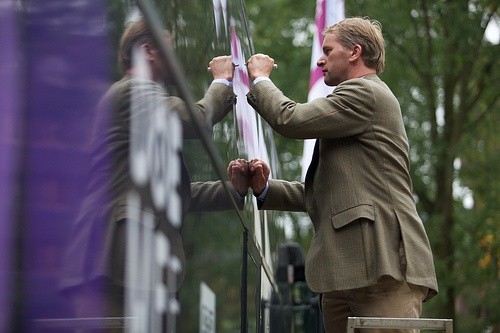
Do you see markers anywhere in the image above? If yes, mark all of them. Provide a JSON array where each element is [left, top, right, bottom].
[[244, 63, 277, 70], [207, 64, 239, 71]]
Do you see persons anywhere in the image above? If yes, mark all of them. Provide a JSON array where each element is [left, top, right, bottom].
[[56, 15, 249, 333], [244, 16, 440, 333]]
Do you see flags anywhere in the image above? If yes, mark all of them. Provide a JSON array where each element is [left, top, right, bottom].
[[229, 26, 271, 179], [300, 0, 345, 189]]
